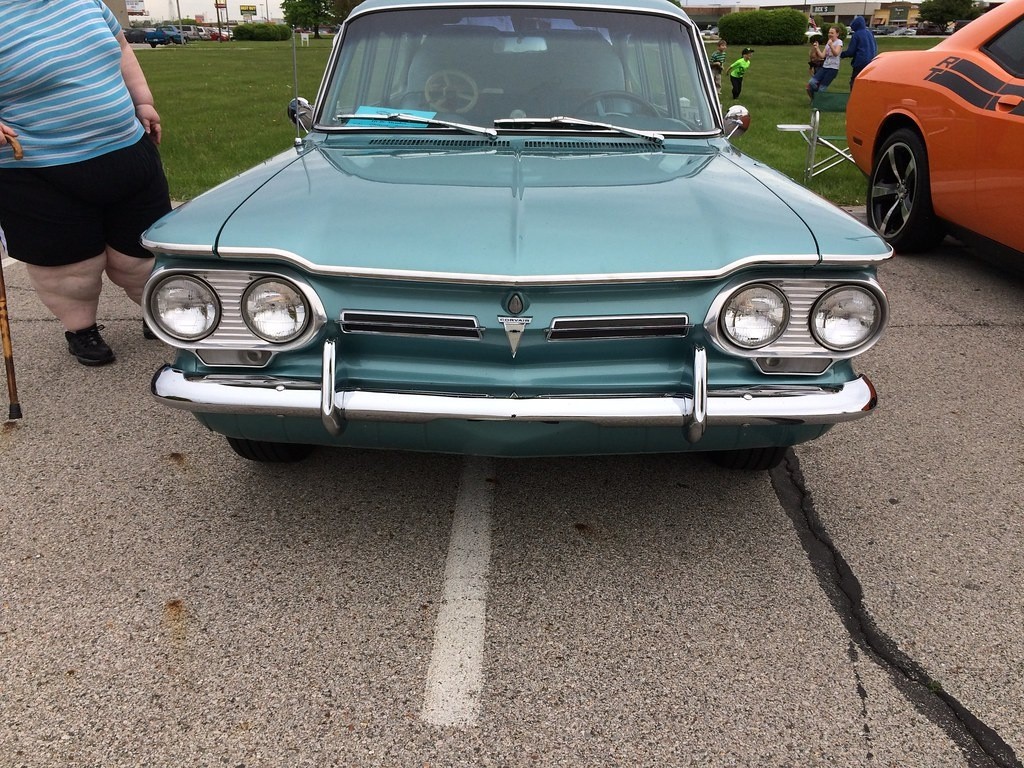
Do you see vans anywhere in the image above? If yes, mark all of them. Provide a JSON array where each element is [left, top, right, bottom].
[[174, 24, 200, 41]]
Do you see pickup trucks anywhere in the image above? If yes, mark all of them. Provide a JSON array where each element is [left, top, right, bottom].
[[145, 25, 187, 48]]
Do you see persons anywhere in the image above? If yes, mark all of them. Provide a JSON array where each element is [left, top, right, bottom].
[[710, 40, 727, 94], [806, 25, 844, 104], [840, 17, 878, 93], [726, 48, 755, 100], [0, 0, 173, 365]]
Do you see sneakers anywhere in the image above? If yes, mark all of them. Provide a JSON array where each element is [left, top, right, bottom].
[[64, 323, 115, 368], [143, 316, 159, 340]]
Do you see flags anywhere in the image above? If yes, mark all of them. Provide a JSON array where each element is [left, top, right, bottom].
[[809, 18, 816, 30]]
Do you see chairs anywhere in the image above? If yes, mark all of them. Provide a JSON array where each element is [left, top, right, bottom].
[[403, 41, 485, 122], [778, 92, 855, 183], [563, 44, 632, 116]]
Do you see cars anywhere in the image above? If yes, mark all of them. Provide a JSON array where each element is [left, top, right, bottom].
[[125, 28, 155, 44], [805, 27, 822, 38], [141, 0, 894, 469], [196, 26, 234, 41], [846, 0, 1024, 252]]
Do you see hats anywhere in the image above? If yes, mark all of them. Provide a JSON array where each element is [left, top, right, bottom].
[[741, 47, 754, 55]]
